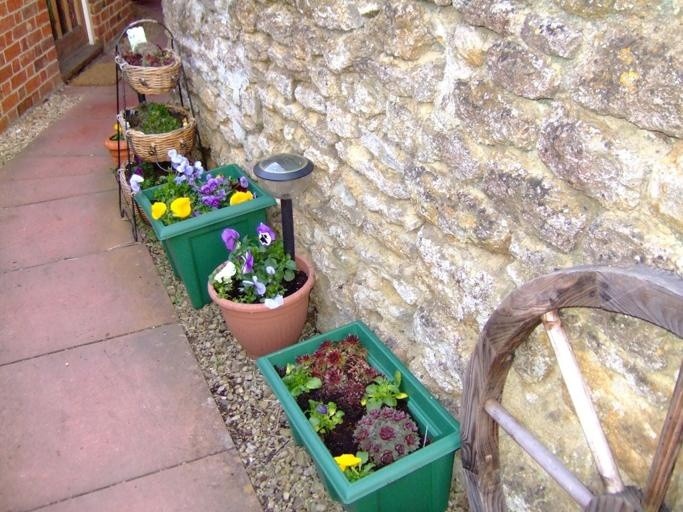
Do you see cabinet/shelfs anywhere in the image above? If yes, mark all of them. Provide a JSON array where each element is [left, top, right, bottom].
[[115, 19, 208, 242]]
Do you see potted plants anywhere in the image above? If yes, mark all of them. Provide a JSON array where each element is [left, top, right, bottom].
[[115, 40, 198, 226]]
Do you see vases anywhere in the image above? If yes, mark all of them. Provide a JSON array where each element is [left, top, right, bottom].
[[104, 133, 131, 166], [131, 164, 278, 311], [251, 319, 462, 511], [207, 253, 317, 362]]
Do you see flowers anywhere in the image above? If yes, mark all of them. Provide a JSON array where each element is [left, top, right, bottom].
[[153, 151, 255, 226], [281, 329, 423, 482], [214, 221, 293, 302], [113, 124, 124, 139]]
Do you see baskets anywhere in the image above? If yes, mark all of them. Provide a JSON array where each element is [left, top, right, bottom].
[[115, 49, 182, 95], [119, 160, 145, 212], [115, 103, 198, 162]]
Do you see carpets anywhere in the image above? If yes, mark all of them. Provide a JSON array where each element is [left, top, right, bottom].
[[74, 63, 121, 86]]
